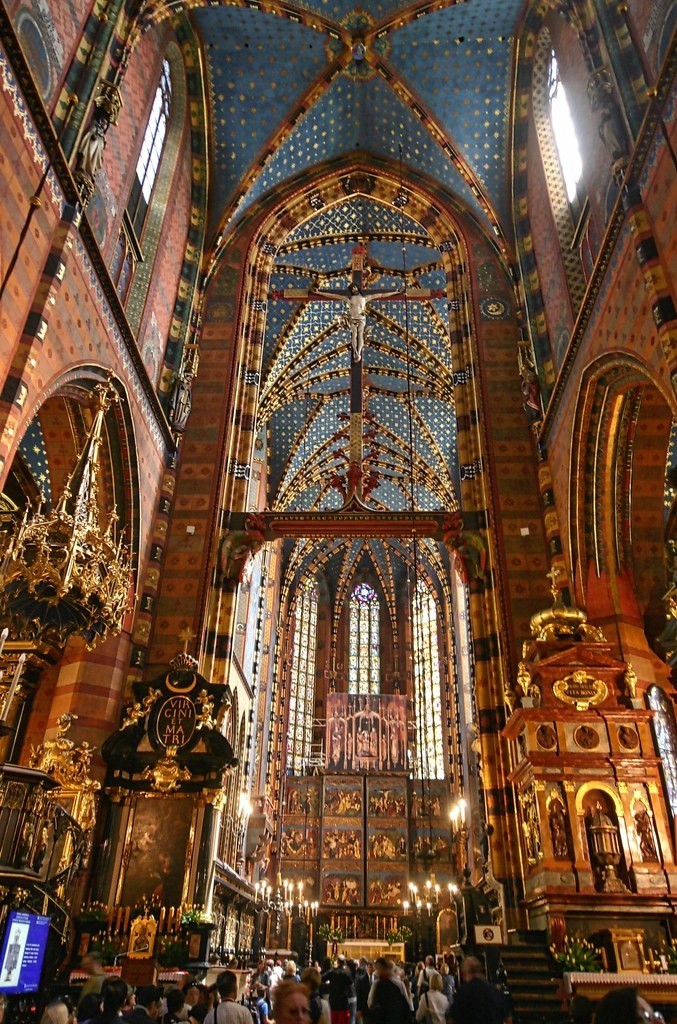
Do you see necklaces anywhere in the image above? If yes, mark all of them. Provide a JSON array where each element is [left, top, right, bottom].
[[223, 999, 233, 1002]]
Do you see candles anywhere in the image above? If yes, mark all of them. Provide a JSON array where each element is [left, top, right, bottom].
[[376, 916, 379, 928], [648, 948, 655, 969], [331, 916, 334, 932], [601, 947, 608, 969], [310, 923, 312, 944], [345, 916, 348, 928], [390, 918, 393, 930], [338, 916, 340, 928], [354, 915, 356, 928], [383, 917, 386, 929], [394, 917, 397, 928], [0, 628, 10, 651], [157, 907, 181, 932], [108, 906, 129, 931], [0, 652, 26, 721]]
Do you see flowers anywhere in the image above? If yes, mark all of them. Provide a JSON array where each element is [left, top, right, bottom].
[[162, 929, 190, 957], [654, 938, 677, 975], [183, 902, 209, 923], [550, 935, 601, 972]]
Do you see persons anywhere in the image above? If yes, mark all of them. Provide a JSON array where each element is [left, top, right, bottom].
[[76, 741, 97, 776], [39, 952, 510, 1024], [593, 987, 665, 1024], [313, 283, 402, 363]]
